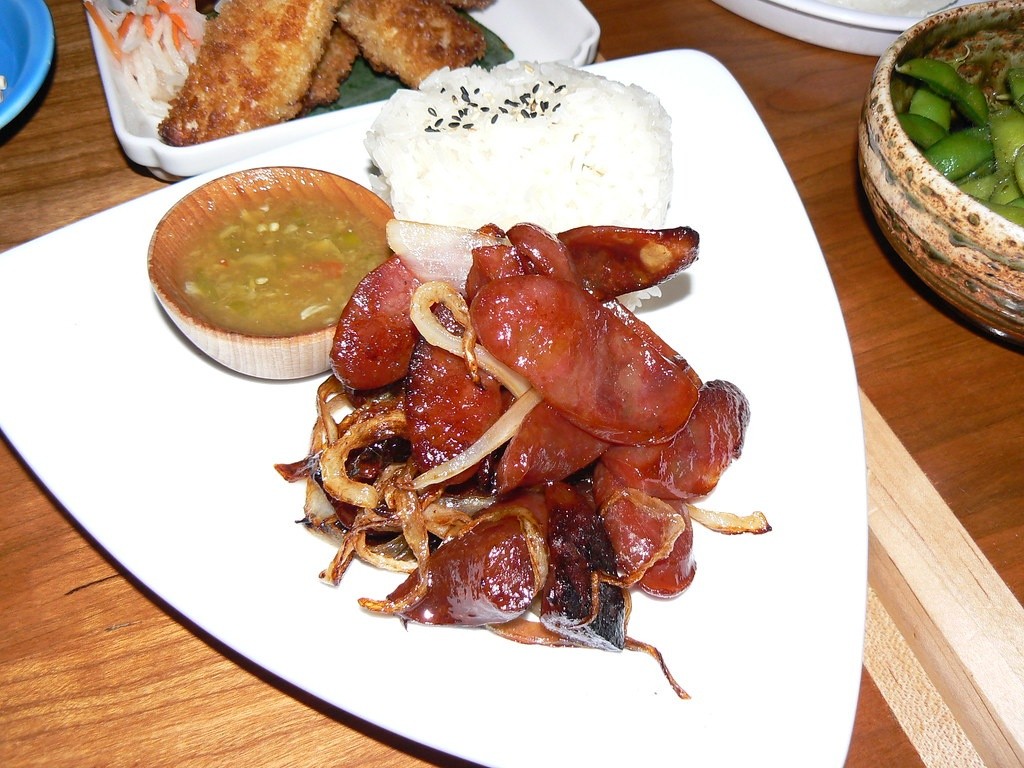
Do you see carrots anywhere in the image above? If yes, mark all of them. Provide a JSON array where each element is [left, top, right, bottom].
[[83, 0, 201, 60]]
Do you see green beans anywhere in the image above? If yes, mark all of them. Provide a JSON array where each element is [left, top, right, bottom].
[[894, 57, 1024, 228]]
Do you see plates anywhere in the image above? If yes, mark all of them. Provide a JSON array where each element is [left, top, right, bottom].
[[85, 0, 601, 182], [0, 0, 55, 130], [0, 47, 867, 768]]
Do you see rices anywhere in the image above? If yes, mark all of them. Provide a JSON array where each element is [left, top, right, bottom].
[[362, 60, 674, 311]]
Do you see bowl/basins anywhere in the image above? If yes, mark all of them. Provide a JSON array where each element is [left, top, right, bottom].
[[859, 0, 1024, 348], [146, 166, 396, 382]]
